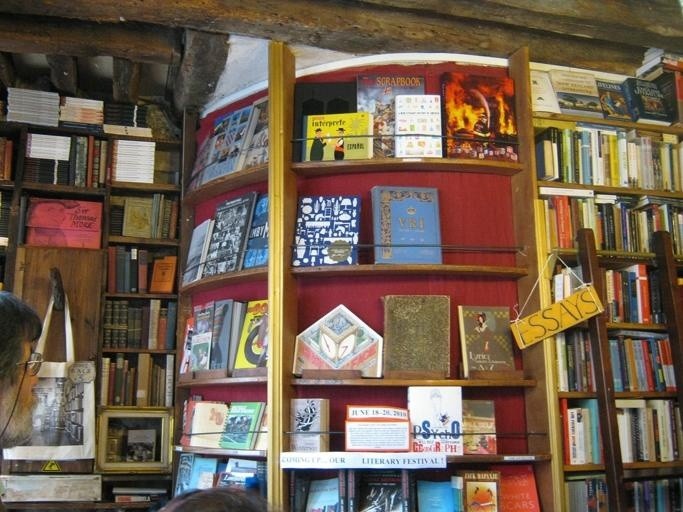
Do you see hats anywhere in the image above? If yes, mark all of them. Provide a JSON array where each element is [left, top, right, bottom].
[[314, 128, 322, 132], [337, 127, 345, 132]]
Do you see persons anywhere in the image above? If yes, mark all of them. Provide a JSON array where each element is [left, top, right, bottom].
[[0, 290, 43, 512]]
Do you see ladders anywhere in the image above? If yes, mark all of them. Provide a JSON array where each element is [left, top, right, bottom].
[[577, 229, 683, 512]]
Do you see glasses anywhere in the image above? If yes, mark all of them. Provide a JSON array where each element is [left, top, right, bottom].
[[15, 351, 43, 376]]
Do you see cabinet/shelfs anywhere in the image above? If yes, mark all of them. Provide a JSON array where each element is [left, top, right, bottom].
[[276, 42, 557, 511], [530, 50, 683, 511], [172, 41, 276, 511], [0, 108, 189, 512]]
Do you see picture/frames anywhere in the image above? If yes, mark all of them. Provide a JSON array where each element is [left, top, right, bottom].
[[95, 406, 174, 472]]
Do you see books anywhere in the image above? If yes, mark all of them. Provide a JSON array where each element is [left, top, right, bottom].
[[0, 83, 183, 503], [525, 45, 681, 511], [171, 74, 544, 512]]
